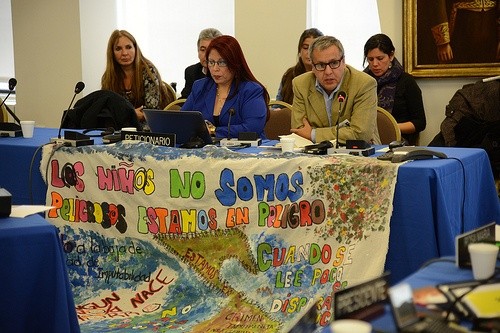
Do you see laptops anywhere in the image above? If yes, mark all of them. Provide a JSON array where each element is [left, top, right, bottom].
[[143, 109, 222, 147]]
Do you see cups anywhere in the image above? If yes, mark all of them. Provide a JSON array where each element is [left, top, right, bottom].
[[467, 242, 499, 281], [20, 121, 36, 138], [330, 319, 373, 333]]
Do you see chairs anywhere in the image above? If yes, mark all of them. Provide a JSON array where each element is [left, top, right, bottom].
[[377, 108, 401, 143], [62, 88, 140, 140], [163, 99, 192, 113], [262, 99, 293, 140]]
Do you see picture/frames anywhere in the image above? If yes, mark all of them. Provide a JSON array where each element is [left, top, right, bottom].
[[403, 1, 500, 78]]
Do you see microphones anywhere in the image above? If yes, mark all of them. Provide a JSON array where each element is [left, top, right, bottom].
[[335, 91, 346, 148], [227, 108, 234, 140], [58, 82, 85, 138], [0, 78, 17, 107]]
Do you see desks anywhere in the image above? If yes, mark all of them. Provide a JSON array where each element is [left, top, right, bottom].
[[0, 132, 500, 301], [319, 255, 499, 333], [0, 213, 83, 332]]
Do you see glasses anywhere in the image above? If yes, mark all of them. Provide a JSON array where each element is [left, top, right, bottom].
[[206, 60, 228, 67], [313, 56, 343, 71]]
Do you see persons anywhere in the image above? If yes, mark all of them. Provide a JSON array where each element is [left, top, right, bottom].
[[173, 29, 224, 107], [102, 29, 169, 128], [290, 36, 381, 146], [362, 34, 427, 148], [269, 29, 323, 110], [180, 36, 270, 144]]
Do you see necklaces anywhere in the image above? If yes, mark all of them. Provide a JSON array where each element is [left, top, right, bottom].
[[216, 87, 226, 102]]
